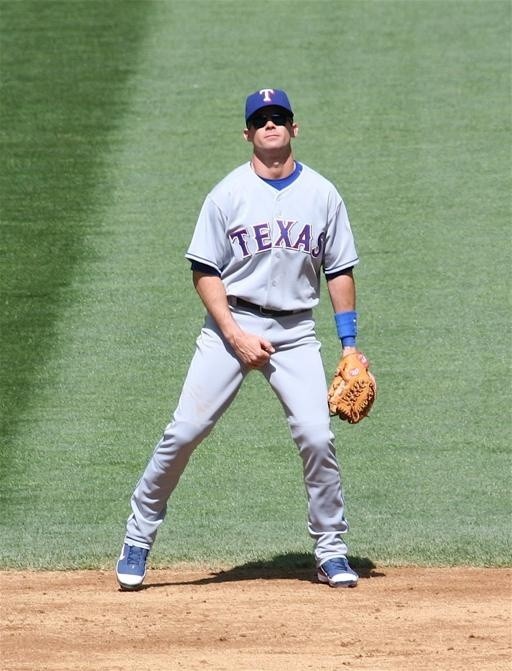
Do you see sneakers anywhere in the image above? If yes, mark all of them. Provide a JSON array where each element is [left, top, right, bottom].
[[116, 543, 148, 588], [317, 556, 359, 588]]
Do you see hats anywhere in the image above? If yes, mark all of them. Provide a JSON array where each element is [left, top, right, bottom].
[[245, 89, 291, 121]]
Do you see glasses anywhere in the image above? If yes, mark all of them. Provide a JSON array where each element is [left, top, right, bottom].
[[253, 112, 287, 129]]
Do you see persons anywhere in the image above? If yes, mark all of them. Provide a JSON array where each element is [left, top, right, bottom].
[[117, 89, 376, 587]]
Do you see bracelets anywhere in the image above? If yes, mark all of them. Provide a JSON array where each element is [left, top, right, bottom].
[[332, 311, 357, 347]]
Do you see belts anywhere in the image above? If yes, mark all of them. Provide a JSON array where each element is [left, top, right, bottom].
[[235, 297, 308, 317]]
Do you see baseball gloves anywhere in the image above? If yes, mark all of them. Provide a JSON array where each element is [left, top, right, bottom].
[[326, 353, 377, 424]]
